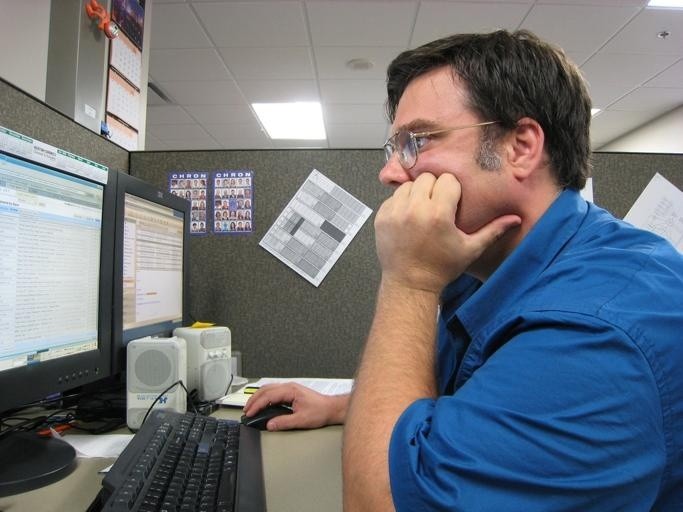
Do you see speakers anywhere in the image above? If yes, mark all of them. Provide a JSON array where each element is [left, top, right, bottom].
[[126, 336, 187, 432], [173, 324, 232, 402]]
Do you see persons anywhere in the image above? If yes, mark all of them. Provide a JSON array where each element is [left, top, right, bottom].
[[171, 180, 205, 233], [243, 28, 682, 512], [215, 179, 250, 232]]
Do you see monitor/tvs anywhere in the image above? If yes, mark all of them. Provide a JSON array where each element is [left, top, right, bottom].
[[0, 126, 118, 498], [75, 170, 191, 418]]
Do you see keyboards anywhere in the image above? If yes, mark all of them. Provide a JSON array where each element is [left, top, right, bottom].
[[85, 408, 266, 512]]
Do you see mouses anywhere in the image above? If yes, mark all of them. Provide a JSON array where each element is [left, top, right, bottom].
[[241, 405, 293, 429]]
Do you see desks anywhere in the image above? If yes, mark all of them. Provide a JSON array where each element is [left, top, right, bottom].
[[0, 375, 345, 511]]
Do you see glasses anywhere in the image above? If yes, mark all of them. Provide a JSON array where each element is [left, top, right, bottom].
[[383, 119, 506, 170]]
